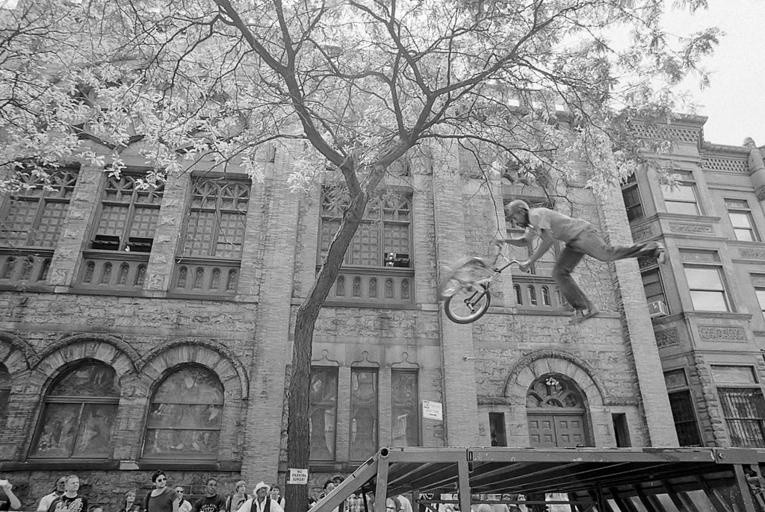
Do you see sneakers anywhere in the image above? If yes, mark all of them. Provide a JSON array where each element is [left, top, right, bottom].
[[655, 242, 667, 265], [568, 304, 599, 326]]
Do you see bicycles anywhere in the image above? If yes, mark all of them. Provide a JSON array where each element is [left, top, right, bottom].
[[433, 239, 531, 325]]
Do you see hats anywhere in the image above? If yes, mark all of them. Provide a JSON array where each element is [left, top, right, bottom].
[[253, 483, 270, 498], [235, 481, 247, 491]]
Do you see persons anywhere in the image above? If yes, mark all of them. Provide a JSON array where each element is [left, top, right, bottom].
[[116, 470, 285, 512], [492, 197, 672, 328], [1, 474, 104, 512], [311, 474, 412, 512], [442, 492, 555, 512]]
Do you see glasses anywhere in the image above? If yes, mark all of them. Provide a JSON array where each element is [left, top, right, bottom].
[[158, 478, 167, 482]]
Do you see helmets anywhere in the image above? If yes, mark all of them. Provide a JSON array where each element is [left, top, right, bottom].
[[504, 199, 530, 220]]
